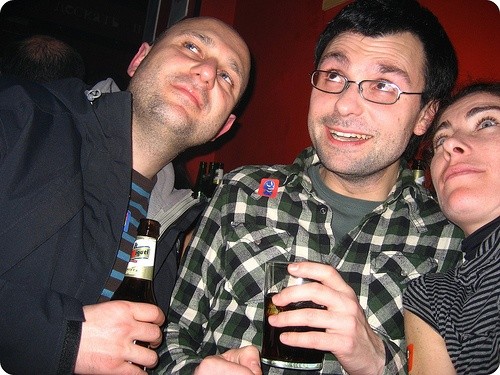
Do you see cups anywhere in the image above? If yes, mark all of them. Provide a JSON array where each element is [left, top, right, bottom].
[[260, 261, 326, 370]]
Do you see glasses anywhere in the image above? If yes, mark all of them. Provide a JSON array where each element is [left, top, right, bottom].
[[310, 70, 423, 105]]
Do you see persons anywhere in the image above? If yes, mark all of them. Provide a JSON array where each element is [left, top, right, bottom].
[[149, 0, 465, 375], [0, 17, 250, 375], [402, 80, 500, 375]]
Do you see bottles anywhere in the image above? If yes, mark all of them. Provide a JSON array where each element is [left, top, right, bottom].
[[194, 162, 224, 201], [109, 219, 161, 371], [411, 160, 427, 190]]
